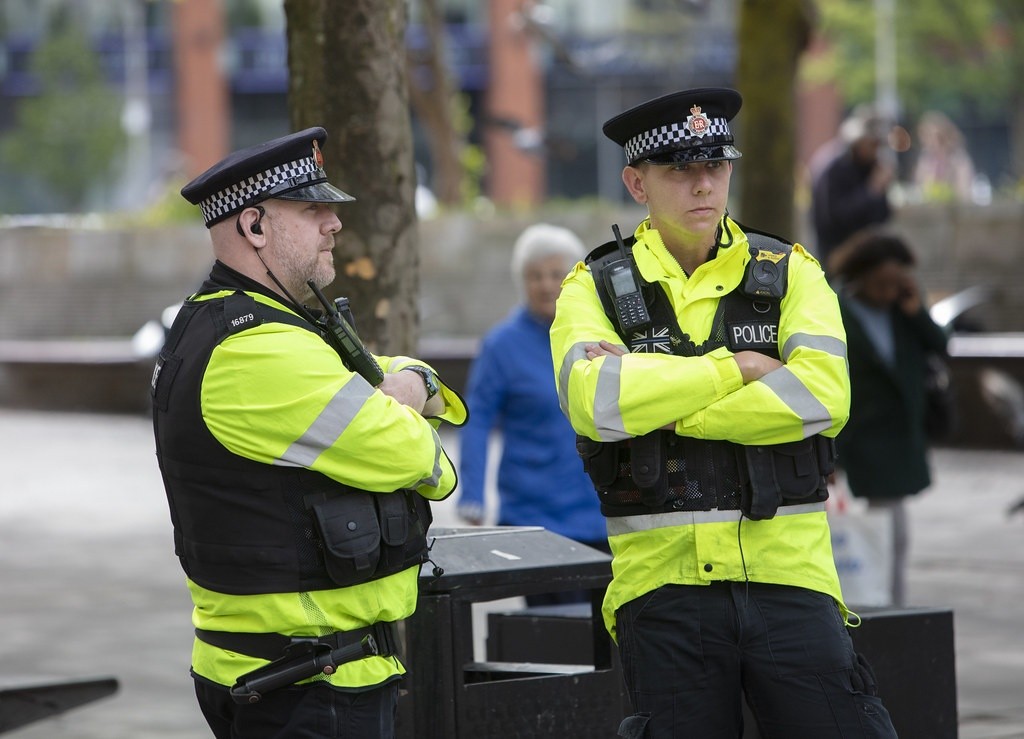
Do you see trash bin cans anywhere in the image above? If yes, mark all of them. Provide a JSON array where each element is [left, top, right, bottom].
[[398, 524, 637, 739]]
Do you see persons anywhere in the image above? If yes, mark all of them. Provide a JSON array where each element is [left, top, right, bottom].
[[458, 222, 611, 608], [809, 108, 896, 264], [823, 231, 949, 610], [550, 88, 899, 739], [150, 126, 470, 739]]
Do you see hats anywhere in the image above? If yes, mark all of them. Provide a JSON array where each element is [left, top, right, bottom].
[[180, 126, 356, 229], [601, 87, 743, 165]]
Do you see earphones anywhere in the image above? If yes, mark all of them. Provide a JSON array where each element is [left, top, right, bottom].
[[236, 207, 264, 235]]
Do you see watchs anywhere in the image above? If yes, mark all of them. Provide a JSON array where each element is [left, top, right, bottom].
[[407, 366, 439, 401]]
